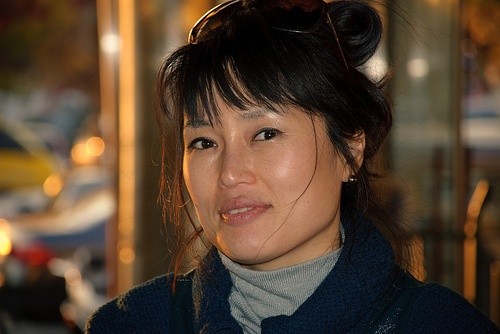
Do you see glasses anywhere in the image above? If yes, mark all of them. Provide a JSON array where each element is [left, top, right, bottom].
[[187, 0, 350, 77]]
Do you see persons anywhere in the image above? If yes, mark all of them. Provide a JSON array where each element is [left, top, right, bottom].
[[84, 0, 500, 334]]
